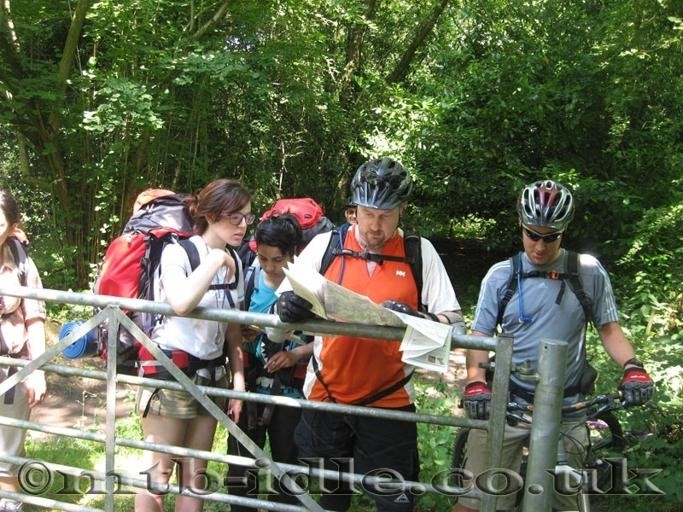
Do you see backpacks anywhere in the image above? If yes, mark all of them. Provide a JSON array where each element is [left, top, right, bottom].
[[233, 196, 337, 388], [93, 189, 239, 372]]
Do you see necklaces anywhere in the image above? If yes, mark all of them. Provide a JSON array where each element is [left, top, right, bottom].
[[203, 239, 229, 349]]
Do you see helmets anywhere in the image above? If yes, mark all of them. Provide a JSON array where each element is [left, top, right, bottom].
[[517, 178, 576, 229], [350, 156, 414, 210], [344, 196, 358, 208]]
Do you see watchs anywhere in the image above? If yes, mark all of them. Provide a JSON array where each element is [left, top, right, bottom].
[[624, 358, 644, 368]]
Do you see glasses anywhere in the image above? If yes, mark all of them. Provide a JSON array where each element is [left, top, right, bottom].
[[519, 223, 565, 244], [224, 211, 257, 226]]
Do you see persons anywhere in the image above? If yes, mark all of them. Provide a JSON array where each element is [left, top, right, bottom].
[[261, 159, 464, 512], [225, 215, 316, 512], [344, 196, 358, 228], [450, 180, 655, 511], [0, 189, 47, 512], [135, 180, 253, 512]]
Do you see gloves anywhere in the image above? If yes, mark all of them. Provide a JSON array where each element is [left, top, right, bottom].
[[276, 291, 316, 323], [381, 297, 425, 319], [462, 382, 494, 421], [618, 369, 654, 408]]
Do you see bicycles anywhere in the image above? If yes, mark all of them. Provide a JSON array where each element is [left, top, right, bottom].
[[448, 392, 626, 512]]
